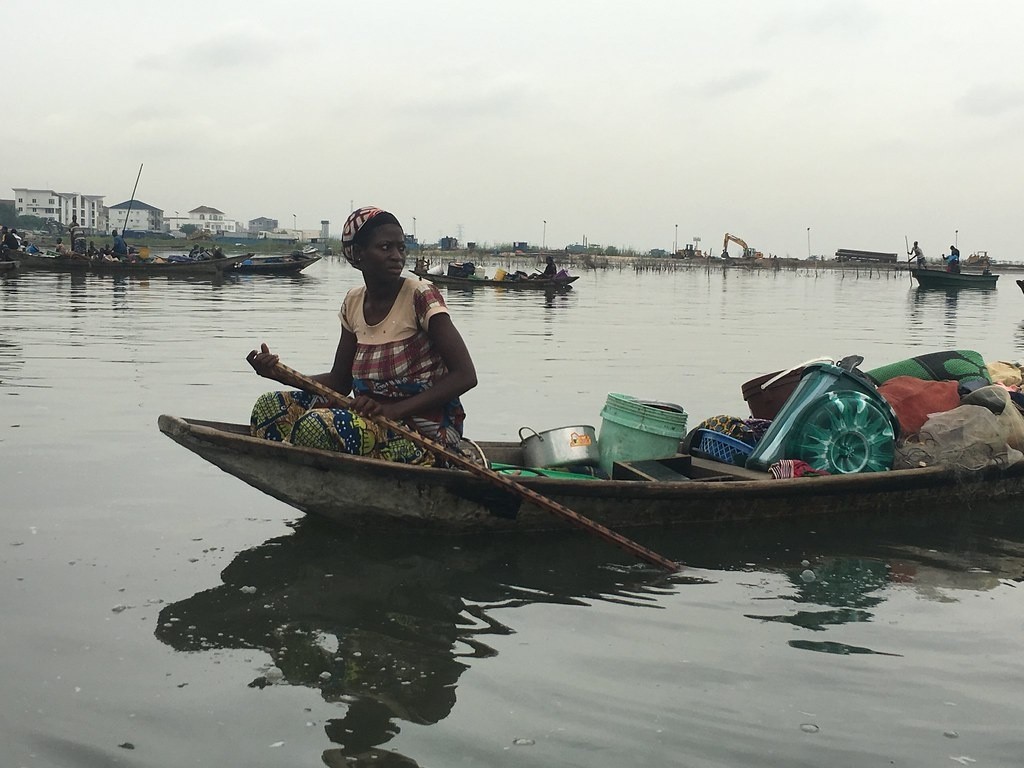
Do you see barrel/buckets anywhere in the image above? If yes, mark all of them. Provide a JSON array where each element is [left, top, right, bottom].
[[427, 265, 444, 276], [598, 392, 688, 479], [475, 269, 485, 279], [745, 363, 900, 476], [494, 269, 506, 281], [140, 248, 149, 258], [26, 245, 39, 254], [741, 357, 834, 421]]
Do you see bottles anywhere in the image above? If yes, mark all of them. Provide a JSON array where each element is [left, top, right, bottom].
[[151, 255, 167, 264]]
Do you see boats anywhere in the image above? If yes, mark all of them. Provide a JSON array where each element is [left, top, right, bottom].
[[408, 269, 579, 287], [909, 268, 1000, 290], [157, 410, 1024, 535], [0, 245, 323, 273]]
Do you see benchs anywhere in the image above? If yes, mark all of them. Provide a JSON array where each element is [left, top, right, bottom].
[[676, 453, 773, 480]]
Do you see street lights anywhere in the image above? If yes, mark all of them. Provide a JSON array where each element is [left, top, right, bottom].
[[293, 214, 297, 230], [543, 220, 547, 247], [807, 228, 811, 257], [675, 224, 678, 251], [413, 217, 416, 239], [955, 230, 958, 248]]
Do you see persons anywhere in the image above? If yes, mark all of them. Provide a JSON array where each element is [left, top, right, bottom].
[[907, 241, 927, 270], [528, 256, 557, 279], [189, 243, 204, 258], [0, 215, 128, 260], [246, 207, 477, 466], [942, 246, 960, 274]]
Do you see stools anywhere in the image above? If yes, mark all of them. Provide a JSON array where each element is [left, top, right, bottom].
[[612, 455, 733, 482]]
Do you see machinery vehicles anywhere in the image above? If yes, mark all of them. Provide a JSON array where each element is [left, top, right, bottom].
[[964, 250, 990, 266], [721, 233, 764, 259]]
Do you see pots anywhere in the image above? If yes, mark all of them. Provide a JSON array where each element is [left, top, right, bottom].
[[519, 425, 600, 469]]
